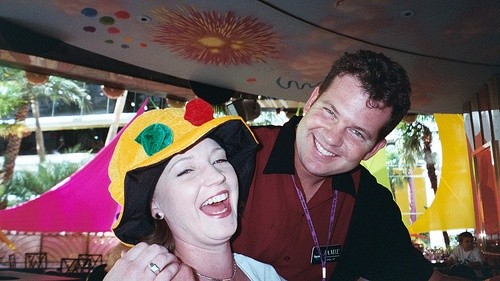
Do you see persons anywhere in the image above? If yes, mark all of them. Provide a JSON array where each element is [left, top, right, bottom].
[[228, 46, 470, 281], [103, 99, 288, 281], [446, 232, 483, 275]]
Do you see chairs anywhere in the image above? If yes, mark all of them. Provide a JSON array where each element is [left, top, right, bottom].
[[83, 264, 107, 280]]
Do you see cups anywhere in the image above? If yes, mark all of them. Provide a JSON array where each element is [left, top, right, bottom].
[[436, 253, 440, 263]]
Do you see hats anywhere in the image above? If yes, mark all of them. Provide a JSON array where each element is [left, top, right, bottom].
[[108, 98, 263, 248]]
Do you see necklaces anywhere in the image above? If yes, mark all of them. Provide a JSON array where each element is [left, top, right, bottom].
[[179, 254, 237, 281]]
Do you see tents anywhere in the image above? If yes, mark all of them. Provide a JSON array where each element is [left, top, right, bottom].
[[0, 98, 149, 266]]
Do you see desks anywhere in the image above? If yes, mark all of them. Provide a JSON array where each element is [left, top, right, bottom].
[[0, 261, 92, 281]]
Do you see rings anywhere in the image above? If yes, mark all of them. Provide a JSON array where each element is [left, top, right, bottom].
[[148, 262, 160, 276]]
